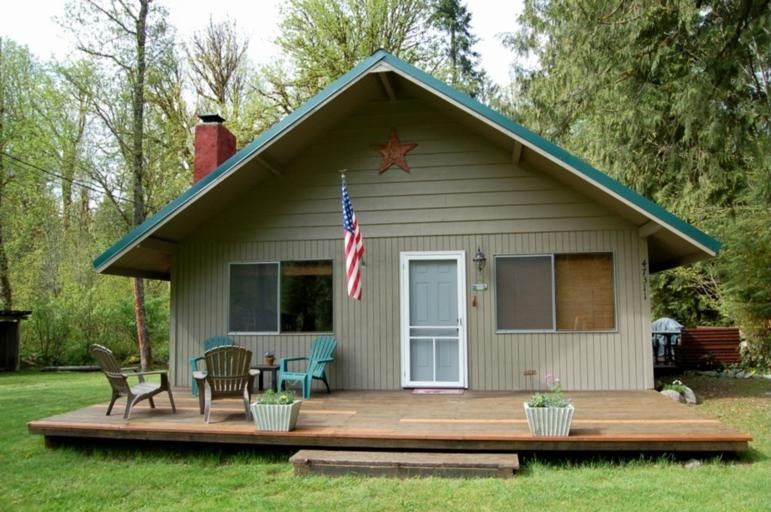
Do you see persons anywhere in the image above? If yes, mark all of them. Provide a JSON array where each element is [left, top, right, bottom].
[[651, 308, 685, 384]]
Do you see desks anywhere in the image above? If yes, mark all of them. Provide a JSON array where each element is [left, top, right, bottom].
[[252, 365, 280, 393]]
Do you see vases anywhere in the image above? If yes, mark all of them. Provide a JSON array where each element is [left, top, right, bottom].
[[523, 402, 575, 436], [250, 400, 301, 432]]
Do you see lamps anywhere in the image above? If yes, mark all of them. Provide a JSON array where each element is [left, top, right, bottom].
[[472, 246, 486, 272]]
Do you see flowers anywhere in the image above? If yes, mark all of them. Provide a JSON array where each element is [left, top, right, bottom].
[[255, 381, 294, 405], [529, 373, 573, 408]]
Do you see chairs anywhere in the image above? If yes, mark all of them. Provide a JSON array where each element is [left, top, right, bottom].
[[190, 335, 261, 423], [277, 337, 338, 400], [89, 344, 176, 421]]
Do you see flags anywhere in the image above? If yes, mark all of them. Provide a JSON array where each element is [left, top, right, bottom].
[[341, 173, 365, 303]]
